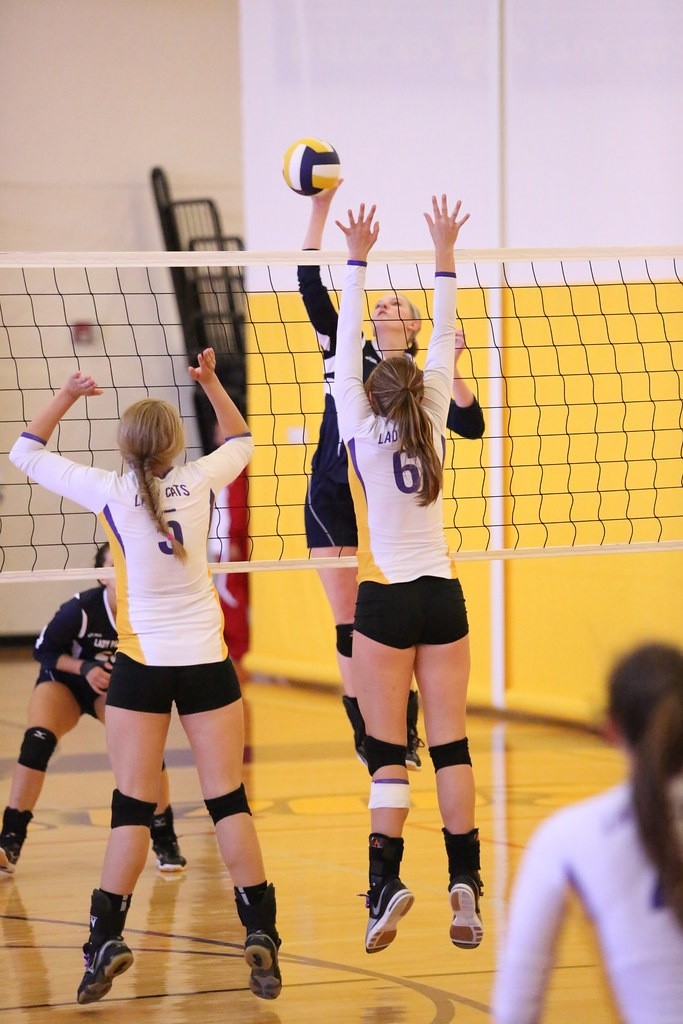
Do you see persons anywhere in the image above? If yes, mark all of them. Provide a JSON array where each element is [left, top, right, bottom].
[[0, 543, 187, 875], [491, 644, 683, 1024], [9, 349, 282, 1005], [297, 181, 484, 954]]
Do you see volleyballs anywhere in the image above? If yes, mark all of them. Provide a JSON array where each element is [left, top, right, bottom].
[[281, 137, 342, 197]]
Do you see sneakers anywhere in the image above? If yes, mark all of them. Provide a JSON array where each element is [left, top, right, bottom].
[[243, 927, 283, 1001], [152, 836, 187, 872], [76, 934, 134, 1005], [404, 725, 425, 771], [0, 831, 22, 873], [448, 874, 484, 951], [357, 874, 415, 954], [353, 733, 369, 768]]
